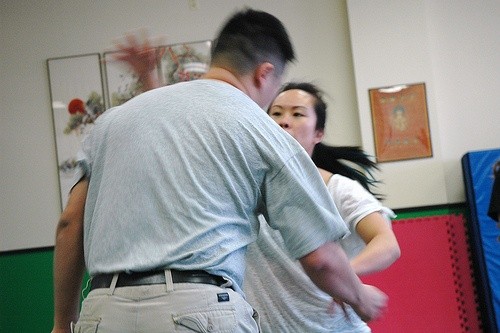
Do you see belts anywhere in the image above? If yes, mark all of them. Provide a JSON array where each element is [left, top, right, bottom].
[[88, 262, 228, 292]]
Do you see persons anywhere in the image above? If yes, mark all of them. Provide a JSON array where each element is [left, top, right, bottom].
[[244, 83, 401, 333], [52, 10, 389, 333]]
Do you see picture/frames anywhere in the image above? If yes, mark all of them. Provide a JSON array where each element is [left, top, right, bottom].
[[104, 47, 160, 109], [158, 40, 213, 86], [47, 52, 106, 213]]
